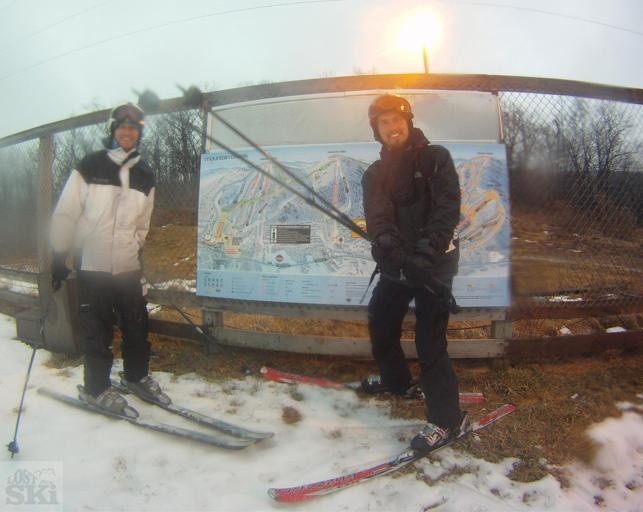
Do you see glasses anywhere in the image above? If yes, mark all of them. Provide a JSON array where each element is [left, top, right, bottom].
[[116, 109, 138, 123], [378, 96, 409, 113]]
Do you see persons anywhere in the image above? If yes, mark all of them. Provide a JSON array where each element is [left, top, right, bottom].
[[47, 101, 160, 415], [355, 93, 460, 455]]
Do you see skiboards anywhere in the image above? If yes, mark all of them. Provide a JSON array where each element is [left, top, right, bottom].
[[257, 365, 515, 503], [37, 379, 275, 450]]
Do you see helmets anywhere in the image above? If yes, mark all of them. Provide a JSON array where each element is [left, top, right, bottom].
[[110, 102, 145, 133], [368, 94, 413, 123]]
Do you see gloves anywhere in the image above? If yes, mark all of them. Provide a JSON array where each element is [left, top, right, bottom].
[[50, 253, 71, 279], [371, 233, 391, 263], [410, 251, 433, 280]]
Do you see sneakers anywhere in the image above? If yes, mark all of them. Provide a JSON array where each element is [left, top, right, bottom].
[[83, 387, 127, 414], [119, 376, 162, 397], [412, 414, 464, 451], [362, 375, 387, 393]]
